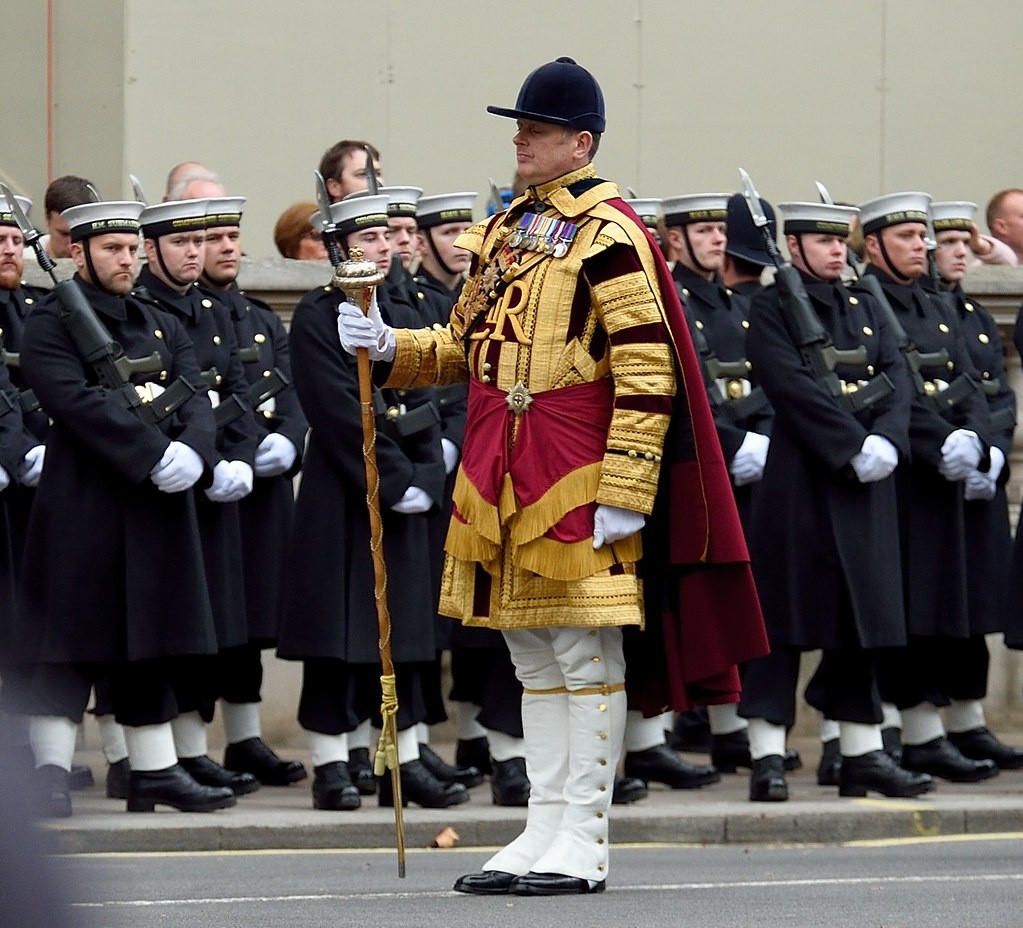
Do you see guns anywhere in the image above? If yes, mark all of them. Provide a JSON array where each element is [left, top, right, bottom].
[[738, 166, 897, 413], [0, 389, 15, 419], [0, 328, 40, 413], [923, 238, 1018, 433], [314, 170, 442, 438], [127, 173, 289, 410], [86, 184, 249, 431], [364, 145, 468, 407], [0, 181, 197, 434], [814, 179, 980, 413], [628, 188, 768, 424]]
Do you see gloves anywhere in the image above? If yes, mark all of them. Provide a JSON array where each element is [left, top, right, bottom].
[[964, 446, 1005, 500], [731, 431, 769, 486], [15, 444, 45, 487], [206, 459, 254, 503], [392, 486, 430, 513], [593, 505, 645, 550], [934, 428, 984, 482], [150, 440, 204, 493], [254, 433, 297, 477], [337, 285, 396, 363], [441, 437, 458, 474], [850, 432, 898, 483]]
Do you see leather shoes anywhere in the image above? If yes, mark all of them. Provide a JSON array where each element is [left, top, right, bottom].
[[105, 755, 129, 798], [31, 735, 533, 816], [611, 724, 1022, 802], [454, 870, 607, 897]]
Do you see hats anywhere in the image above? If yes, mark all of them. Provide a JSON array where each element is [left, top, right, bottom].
[[624, 191, 977, 269], [486, 57, 605, 133], [415, 191, 478, 228], [342, 186, 422, 217], [206, 196, 248, 228], [308, 194, 391, 243], [0, 194, 32, 226], [59, 201, 146, 244], [141, 198, 210, 236]]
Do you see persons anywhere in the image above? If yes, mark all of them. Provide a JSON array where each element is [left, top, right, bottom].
[[613, 189, 1023, 801], [0, 140, 532, 817], [334, 55, 768, 893]]
